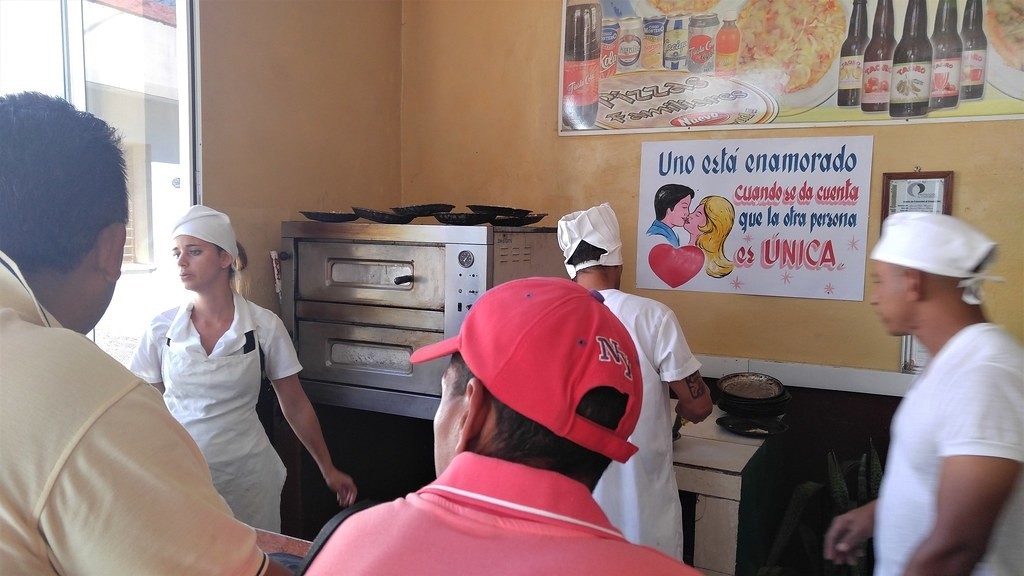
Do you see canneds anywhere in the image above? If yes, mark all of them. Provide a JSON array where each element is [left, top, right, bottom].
[[599, 13, 720, 79]]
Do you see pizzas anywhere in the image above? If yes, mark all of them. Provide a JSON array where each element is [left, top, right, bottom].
[[646, 0, 720, 15], [736, 0, 846, 93], [983, 0, 1024, 72]]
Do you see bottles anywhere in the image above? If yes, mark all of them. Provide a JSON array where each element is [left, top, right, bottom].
[[862, 0, 895, 111], [715, 10, 740, 77], [838, 0, 870, 107], [889, 0, 932, 117], [930, 0, 963, 108], [960, 0, 987, 99], [562, 0, 602, 130]]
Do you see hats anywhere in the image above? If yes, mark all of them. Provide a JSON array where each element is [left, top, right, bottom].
[[869, 211, 1006, 305], [409, 277, 643, 464], [171, 204, 238, 264], [557, 202, 624, 279]]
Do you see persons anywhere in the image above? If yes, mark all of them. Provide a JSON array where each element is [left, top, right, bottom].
[[826, 212, 1024, 575], [0, 91, 296, 576], [557, 203, 713, 562], [129, 205, 357, 533], [305, 277, 703, 576]]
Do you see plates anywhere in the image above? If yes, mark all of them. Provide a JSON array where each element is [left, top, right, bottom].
[[713, 0, 851, 116], [717, 370, 792, 436], [299, 202, 550, 226], [980, 0, 1024, 100]]
